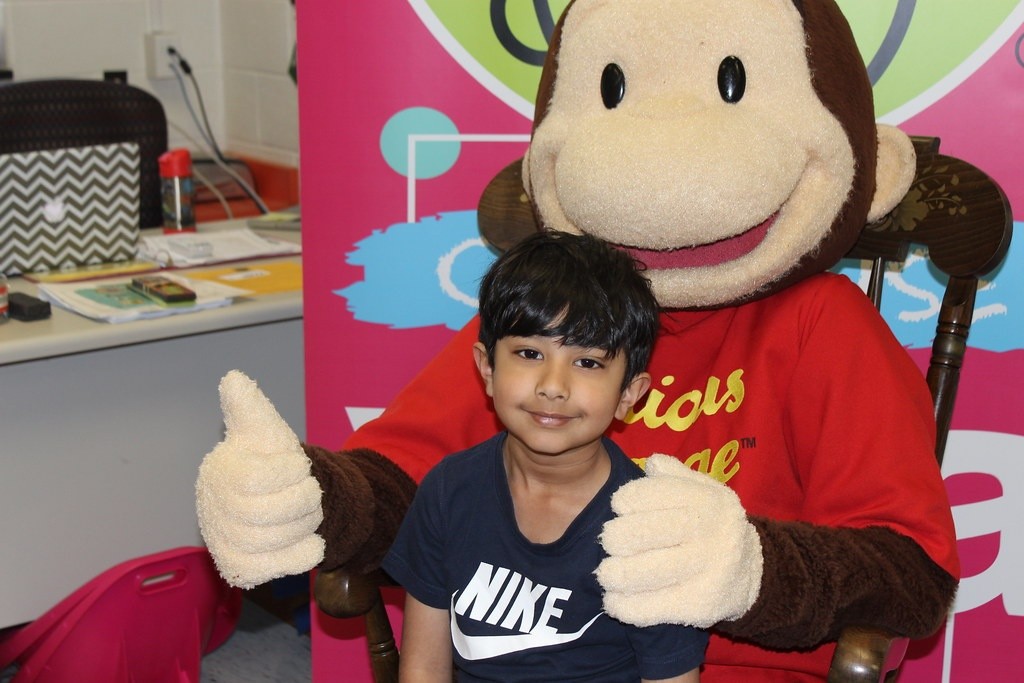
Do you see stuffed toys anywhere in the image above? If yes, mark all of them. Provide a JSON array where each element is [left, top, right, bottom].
[[194, 0, 961, 683]]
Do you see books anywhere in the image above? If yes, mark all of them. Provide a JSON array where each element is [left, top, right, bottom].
[[36, 271, 253, 324], [139, 227, 302, 269]]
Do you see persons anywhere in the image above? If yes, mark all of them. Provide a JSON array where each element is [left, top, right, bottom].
[[380, 228, 709, 682]]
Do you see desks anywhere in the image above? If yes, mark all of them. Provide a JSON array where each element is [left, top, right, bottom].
[[2, 215, 306, 627]]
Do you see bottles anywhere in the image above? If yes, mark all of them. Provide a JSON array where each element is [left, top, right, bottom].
[[0, 272, 9, 325]]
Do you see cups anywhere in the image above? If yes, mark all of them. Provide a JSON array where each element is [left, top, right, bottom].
[[161, 147, 196, 235]]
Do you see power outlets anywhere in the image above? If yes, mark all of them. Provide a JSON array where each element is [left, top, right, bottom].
[[146, 31, 185, 83]]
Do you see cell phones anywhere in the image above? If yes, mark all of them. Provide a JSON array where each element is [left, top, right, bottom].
[[132, 276, 196, 303]]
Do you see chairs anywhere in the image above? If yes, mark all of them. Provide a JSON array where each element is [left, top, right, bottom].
[[315, 135, 1014, 683], [0, 77, 169, 228], [0, 545, 226, 683]]
